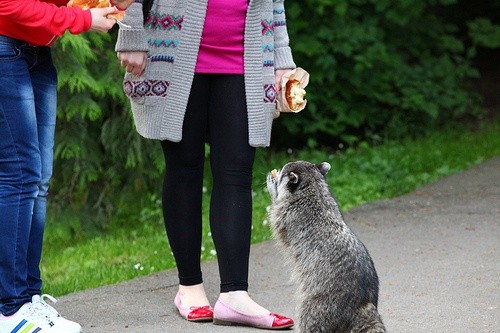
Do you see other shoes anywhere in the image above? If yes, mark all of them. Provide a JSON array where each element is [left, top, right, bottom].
[[213, 297, 295, 329], [174, 289, 214, 322]]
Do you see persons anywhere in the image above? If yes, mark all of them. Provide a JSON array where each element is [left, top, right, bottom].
[[114, 0, 295, 330], [0, 0, 117, 333]]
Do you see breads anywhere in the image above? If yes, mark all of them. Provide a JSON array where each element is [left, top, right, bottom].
[[285, 79, 306, 110]]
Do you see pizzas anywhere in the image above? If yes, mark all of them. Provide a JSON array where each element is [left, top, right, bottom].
[[66, 0, 127, 21]]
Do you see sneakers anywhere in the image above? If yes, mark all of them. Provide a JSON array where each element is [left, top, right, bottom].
[[31, 293, 82, 331], [0, 301, 83, 333]]
